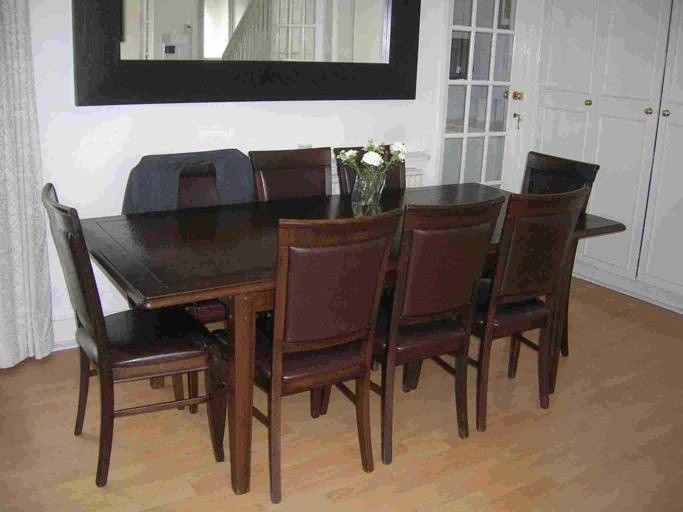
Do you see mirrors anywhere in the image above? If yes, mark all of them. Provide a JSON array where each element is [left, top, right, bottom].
[[71, 0, 420, 105]]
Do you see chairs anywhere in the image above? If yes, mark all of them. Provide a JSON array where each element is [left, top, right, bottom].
[[41, 182, 226, 487], [248, 147, 332, 201], [334, 143, 405, 193], [507, 151, 600, 379], [320, 196, 507, 463], [212, 208, 401, 504], [431, 185, 590, 431], [120, 149, 266, 413]]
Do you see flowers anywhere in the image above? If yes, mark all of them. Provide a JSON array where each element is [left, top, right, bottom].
[[336, 133, 410, 213]]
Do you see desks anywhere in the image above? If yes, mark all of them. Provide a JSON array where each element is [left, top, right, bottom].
[[80, 182, 625, 495]]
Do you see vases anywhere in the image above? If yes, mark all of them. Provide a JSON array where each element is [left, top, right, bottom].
[[349, 171, 386, 218]]
